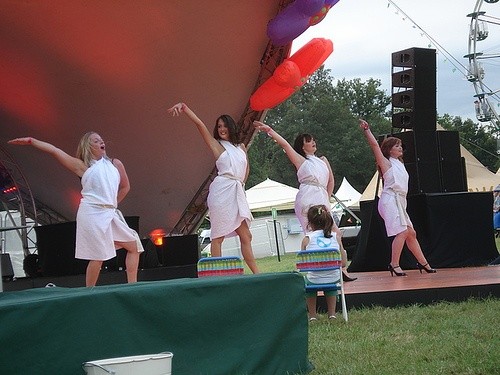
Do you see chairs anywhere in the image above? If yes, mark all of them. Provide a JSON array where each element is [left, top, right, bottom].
[[295, 248, 349, 323], [196, 256, 244, 278]]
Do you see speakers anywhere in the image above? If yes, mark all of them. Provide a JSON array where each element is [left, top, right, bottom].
[[438, 131, 465, 192], [1, 253, 14, 278], [162, 234, 202, 267], [138, 239, 159, 269], [379, 130, 443, 195], [391, 47, 436, 130]]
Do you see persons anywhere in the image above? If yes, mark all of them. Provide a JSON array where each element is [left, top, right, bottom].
[[7, 130, 146, 288], [358, 117, 439, 277], [492, 183, 500, 256], [251, 120, 359, 282], [299, 204, 345, 322], [167, 103, 261, 275]]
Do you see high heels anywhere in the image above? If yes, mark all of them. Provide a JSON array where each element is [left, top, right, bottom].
[[417, 262, 436, 274], [388, 264, 406, 276]]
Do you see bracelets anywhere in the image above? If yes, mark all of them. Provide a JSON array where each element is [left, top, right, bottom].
[[181, 104, 187, 112], [266, 127, 272, 135], [28, 137, 33, 145], [364, 127, 369, 131]]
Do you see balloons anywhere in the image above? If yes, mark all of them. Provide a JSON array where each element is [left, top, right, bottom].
[[265, 0, 340, 48], [248, 37, 334, 112]]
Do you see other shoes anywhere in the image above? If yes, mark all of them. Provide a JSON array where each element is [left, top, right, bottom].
[[310, 318, 317, 321], [342, 272, 357, 282], [329, 316, 336, 319]]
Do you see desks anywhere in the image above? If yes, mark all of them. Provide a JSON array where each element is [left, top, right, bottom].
[[344, 190, 500, 272]]
